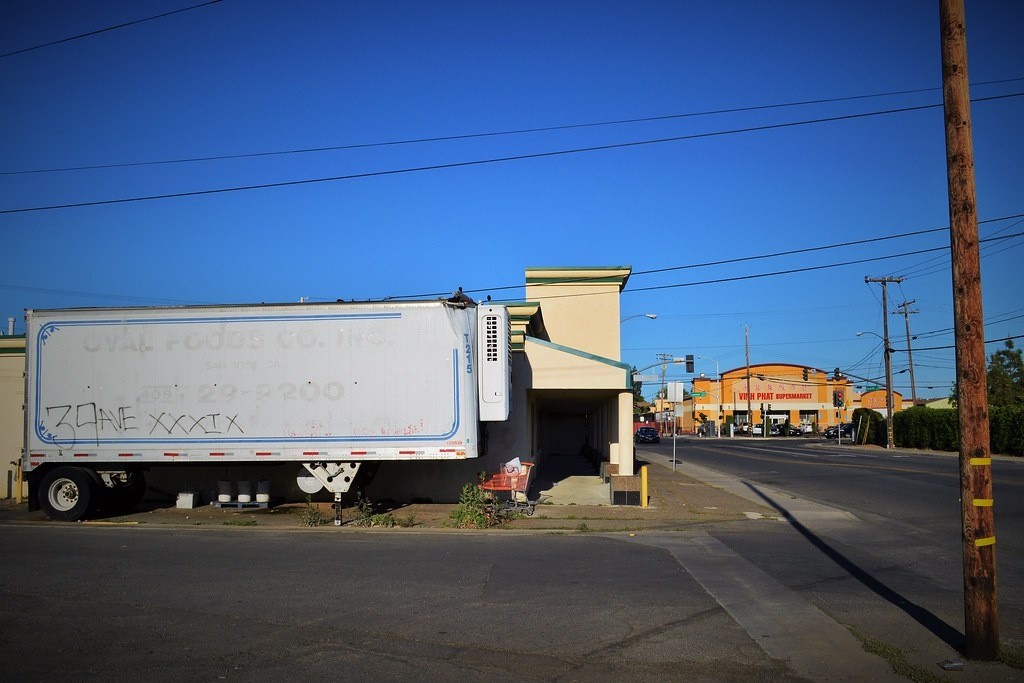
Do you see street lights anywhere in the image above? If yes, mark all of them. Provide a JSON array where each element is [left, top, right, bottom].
[[697, 355, 721, 436], [857, 331, 898, 447]]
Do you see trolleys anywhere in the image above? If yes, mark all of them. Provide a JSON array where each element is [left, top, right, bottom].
[[477, 461, 534, 519]]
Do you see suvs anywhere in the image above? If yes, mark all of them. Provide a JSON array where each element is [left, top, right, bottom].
[[824, 422, 855, 439]]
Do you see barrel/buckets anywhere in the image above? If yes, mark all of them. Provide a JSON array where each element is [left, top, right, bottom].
[[217, 480, 231, 502], [255, 478, 271, 503], [237, 480, 251, 502]]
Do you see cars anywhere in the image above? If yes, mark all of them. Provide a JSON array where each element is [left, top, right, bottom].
[[799, 422, 813, 434], [732, 421, 781, 437], [634, 426, 660, 445], [776, 423, 802, 436]]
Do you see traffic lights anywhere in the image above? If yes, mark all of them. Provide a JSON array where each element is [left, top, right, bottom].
[[803, 366, 808, 381], [760, 403, 763, 410], [768, 404, 772, 411], [834, 367, 840, 381]]
